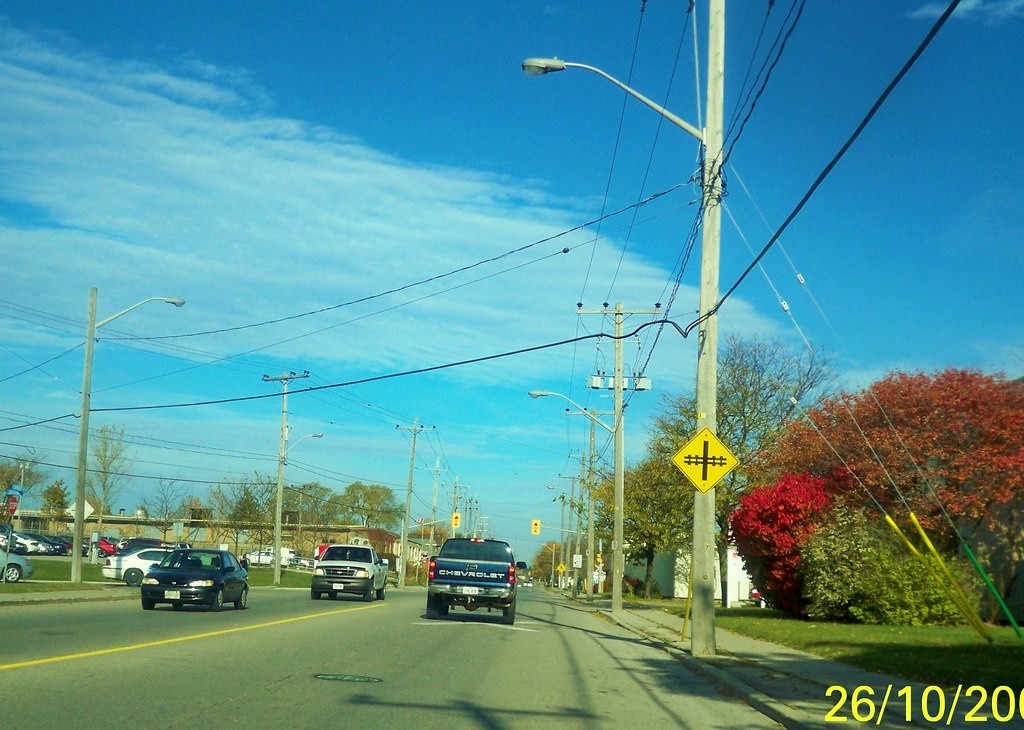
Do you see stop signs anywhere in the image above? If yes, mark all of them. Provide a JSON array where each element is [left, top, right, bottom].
[[9, 498, 18, 515]]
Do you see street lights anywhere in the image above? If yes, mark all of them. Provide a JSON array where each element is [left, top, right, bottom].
[[520, 59, 718, 661], [273, 433, 324, 588], [547, 486, 585, 600], [528, 390, 624, 617], [72, 296, 186, 583], [18, 463, 29, 521]]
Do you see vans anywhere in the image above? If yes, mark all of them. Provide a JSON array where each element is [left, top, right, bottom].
[[161, 542, 191, 549], [267, 548, 295, 559]]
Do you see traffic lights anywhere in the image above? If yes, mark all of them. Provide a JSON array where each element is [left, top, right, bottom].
[[531, 520, 541, 536], [595, 553, 601, 563], [451, 514, 461, 528]]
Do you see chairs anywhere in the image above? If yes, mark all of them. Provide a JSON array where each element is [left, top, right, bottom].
[[211, 557, 220, 567]]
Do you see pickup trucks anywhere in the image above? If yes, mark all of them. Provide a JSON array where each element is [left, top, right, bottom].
[[312, 545, 389, 602], [426, 539, 528, 627]]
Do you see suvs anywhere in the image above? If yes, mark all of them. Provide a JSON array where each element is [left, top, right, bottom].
[[122, 538, 162, 555]]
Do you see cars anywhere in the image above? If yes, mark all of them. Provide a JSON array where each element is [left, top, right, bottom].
[[100, 548, 197, 586], [522, 579, 534, 588], [105, 536, 130, 550], [292, 558, 309, 567], [0, 548, 33, 583], [0, 524, 102, 557], [141, 548, 248, 611], [82, 537, 115, 556], [238, 551, 289, 569]]
